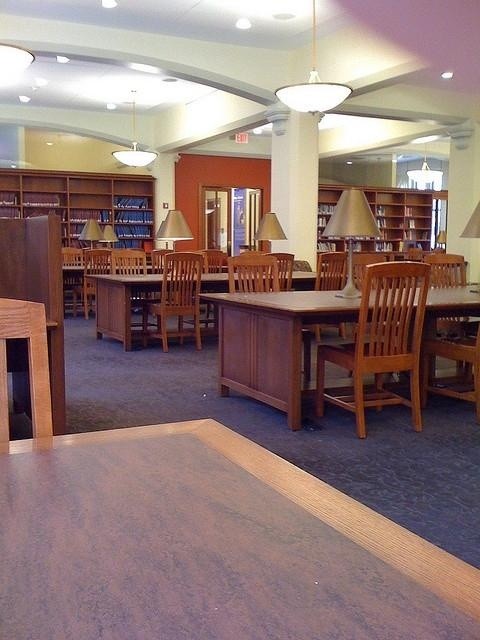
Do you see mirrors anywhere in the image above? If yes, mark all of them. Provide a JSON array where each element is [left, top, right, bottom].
[[431, 197, 447, 249], [202, 188, 230, 255]]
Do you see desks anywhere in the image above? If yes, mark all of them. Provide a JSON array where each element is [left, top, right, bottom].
[[85, 272, 357, 351], [0, 419, 479, 639], [63, 266, 228, 319], [197, 286, 480, 429]]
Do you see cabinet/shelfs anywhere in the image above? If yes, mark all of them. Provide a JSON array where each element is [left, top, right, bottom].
[[317, 184, 432, 255], [0, 168, 157, 263]]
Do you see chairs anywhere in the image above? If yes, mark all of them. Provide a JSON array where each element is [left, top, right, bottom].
[[315, 252, 347, 342], [263, 253, 295, 291], [346, 254, 398, 343], [316, 261, 431, 438], [178, 251, 218, 329], [421, 322, 479, 424], [72, 249, 112, 320], [62, 247, 83, 295], [142, 252, 205, 353], [111, 250, 161, 332], [151, 250, 175, 318], [227, 255, 312, 389], [0, 299, 53, 443], [424, 254, 470, 382], [202, 249, 224, 329]]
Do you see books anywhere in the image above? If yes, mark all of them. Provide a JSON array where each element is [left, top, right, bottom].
[[71, 225, 104, 236], [115, 211, 153, 224], [0, 192, 17, 205], [114, 198, 148, 209], [318, 231, 340, 239], [352, 242, 361, 251], [378, 218, 386, 228], [317, 242, 335, 252], [27, 209, 66, 221], [345, 236, 370, 240], [377, 230, 387, 240], [63, 240, 67, 247], [376, 242, 403, 252], [404, 230, 427, 240], [23, 193, 59, 206], [408, 220, 414, 228], [62, 224, 67, 236], [71, 210, 111, 222], [115, 240, 142, 248], [318, 204, 336, 212], [71, 240, 91, 249], [378, 206, 385, 216], [115, 226, 150, 237], [319, 217, 326, 226], [93, 242, 112, 248], [407, 208, 412, 216], [0, 208, 20, 219]]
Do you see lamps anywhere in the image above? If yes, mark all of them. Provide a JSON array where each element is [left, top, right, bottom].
[[322, 190, 384, 299], [78, 219, 106, 266], [253, 213, 288, 275], [157, 210, 195, 277], [155, 221, 169, 249], [407, 148, 443, 185], [274, 0, 353, 113], [112, 102, 158, 167], [98, 225, 119, 249], [437, 231, 446, 249]]
